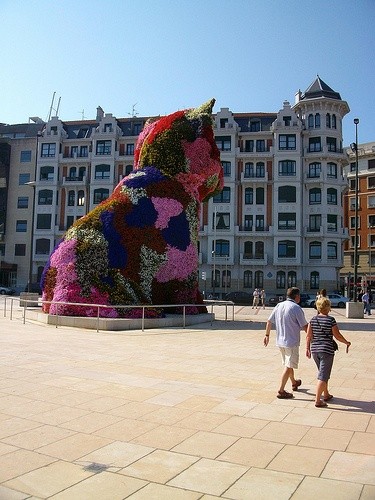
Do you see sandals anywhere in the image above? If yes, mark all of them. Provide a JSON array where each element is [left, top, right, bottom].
[[276, 390, 293, 399], [291, 379, 302, 391]]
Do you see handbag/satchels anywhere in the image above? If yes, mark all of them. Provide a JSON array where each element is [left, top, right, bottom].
[[332, 339, 339, 351]]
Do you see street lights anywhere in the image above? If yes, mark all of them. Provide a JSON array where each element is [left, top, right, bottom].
[[24, 132, 44, 283], [211, 206, 216, 300], [345, 118, 364, 319]]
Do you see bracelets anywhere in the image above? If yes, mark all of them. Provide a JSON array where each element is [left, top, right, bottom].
[[265, 335, 269, 336]]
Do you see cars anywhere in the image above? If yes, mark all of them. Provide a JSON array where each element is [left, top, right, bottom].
[[306, 294, 349, 308], [0, 284, 16, 295], [224, 291, 310, 307]]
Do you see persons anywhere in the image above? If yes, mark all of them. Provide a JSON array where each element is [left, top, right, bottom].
[[263, 287, 309, 399], [252, 288, 260, 309], [358, 290, 373, 315], [306, 296, 351, 407], [260, 290, 266, 310]]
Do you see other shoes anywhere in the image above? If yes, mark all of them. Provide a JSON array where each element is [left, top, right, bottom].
[[315, 400, 326, 407], [323, 394, 333, 401], [256, 307, 257, 309], [252, 307, 254, 309], [363, 312, 372, 316]]
